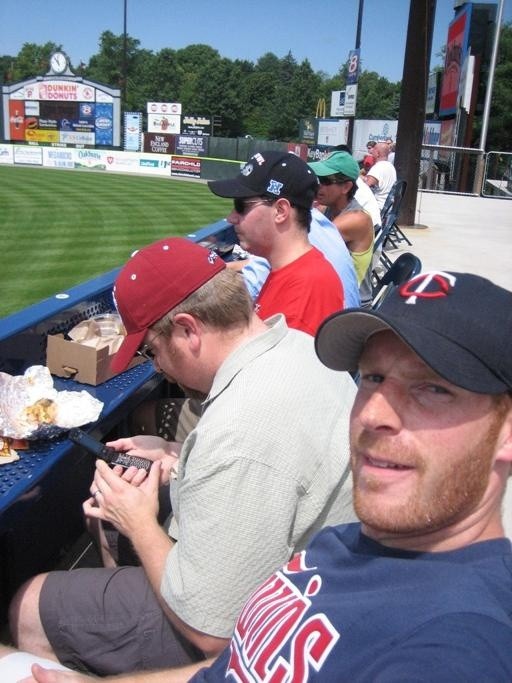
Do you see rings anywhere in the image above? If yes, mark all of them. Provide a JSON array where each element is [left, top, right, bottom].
[[92, 490, 102, 498]]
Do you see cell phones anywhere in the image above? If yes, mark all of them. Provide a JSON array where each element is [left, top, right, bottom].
[[68, 426, 153, 475]]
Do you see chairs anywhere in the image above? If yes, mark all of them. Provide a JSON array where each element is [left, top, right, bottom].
[[382, 180, 412, 247], [373, 250, 422, 303], [380, 188, 399, 249], [379, 200, 394, 272], [369, 212, 398, 288]]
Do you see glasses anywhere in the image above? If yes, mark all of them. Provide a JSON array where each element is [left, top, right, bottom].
[[229, 197, 280, 217], [368, 147, 372, 149], [134, 318, 174, 363], [317, 174, 347, 188]]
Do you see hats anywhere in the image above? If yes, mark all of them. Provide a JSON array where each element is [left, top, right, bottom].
[[109, 238, 227, 376], [314, 270, 512, 395], [207, 150, 321, 212], [307, 149, 360, 183]]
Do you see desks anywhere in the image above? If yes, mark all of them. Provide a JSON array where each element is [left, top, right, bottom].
[[0, 221, 241, 515]]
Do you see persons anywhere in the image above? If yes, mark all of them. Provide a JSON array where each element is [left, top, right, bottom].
[[207, 151, 344, 337], [360, 155, 375, 176], [306, 151, 374, 311], [11, 236, 360, 677], [225, 207, 360, 308], [360, 143, 397, 217], [388, 143, 396, 165], [366, 141, 377, 154], [0, 271, 512, 683], [386, 138, 393, 148]]
